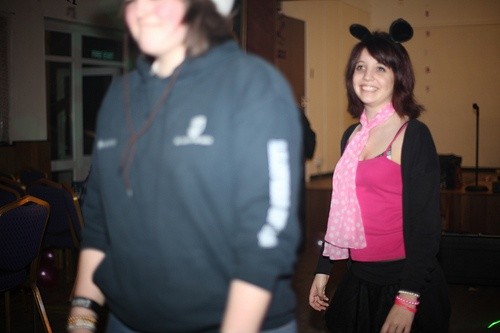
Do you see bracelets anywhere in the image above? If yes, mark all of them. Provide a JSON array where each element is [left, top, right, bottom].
[[67, 297, 102, 330], [394, 290, 420, 313]]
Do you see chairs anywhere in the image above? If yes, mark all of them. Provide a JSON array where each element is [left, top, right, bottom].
[[0, 141, 83, 333]]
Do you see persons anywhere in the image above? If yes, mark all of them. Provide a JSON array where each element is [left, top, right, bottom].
[[308, 32, 449, 333], [66, 0, 306, 333]]
